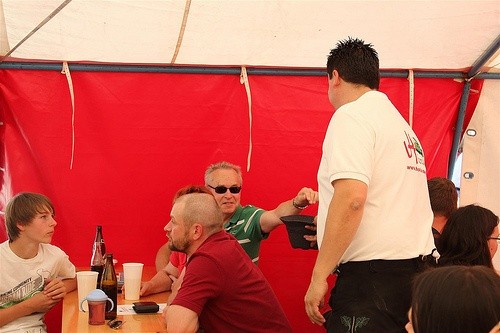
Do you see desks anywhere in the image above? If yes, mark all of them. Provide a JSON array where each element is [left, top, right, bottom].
[[62, 285, 172, 333]]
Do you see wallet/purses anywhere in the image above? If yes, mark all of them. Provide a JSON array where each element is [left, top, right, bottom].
[[133, 301, 160, 313]]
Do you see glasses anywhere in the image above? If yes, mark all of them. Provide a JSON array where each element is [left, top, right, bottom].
[[487, 235, 500, 242], [207, 185, 241, 194]]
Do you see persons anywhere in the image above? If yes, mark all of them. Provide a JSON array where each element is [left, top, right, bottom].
[[405, 177, 500, 333], [203, 163, 320, 265], [0, 192, 78, 333], [119, 184, 294, 333], [304, 34, 440, 333]]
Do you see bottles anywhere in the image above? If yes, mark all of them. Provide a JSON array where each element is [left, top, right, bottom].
[[80, 289, 114, 325], [100, 253, 117, 320], [90, 242, 103, 289], [92, 226, 106, 256]]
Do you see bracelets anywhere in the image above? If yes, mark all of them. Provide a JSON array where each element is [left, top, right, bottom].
[[292, 198, 309, 210]]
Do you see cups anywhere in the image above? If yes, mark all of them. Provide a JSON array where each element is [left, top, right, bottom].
[[123, 263, 144, 300], [76, 271, 99, 311]]
